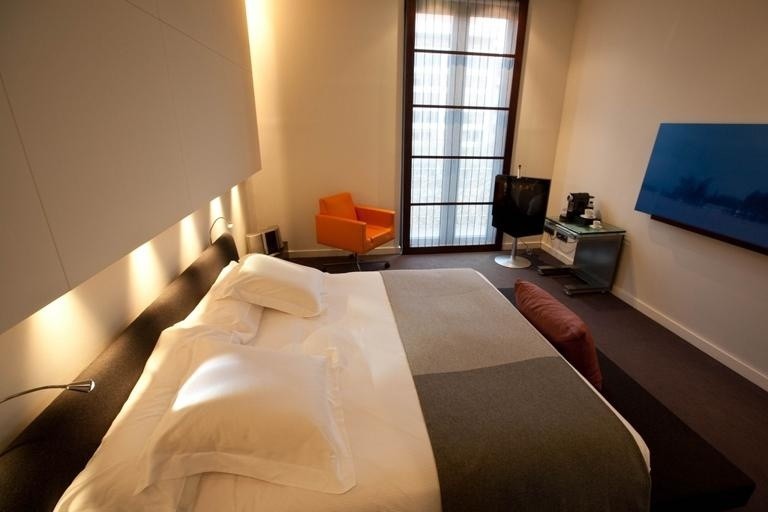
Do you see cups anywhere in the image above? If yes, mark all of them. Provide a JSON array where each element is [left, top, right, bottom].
[[585, 209, 593, 217], [593, 220, 600, 227]]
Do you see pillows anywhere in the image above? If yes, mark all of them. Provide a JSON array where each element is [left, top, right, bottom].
[[515, 278, 610, 396], [213, 253, 332, 320], [129, 336, 361, 497]]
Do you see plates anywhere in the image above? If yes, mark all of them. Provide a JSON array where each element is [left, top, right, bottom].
[[589, 225, 603, 229], [580, 214, 597, 219]]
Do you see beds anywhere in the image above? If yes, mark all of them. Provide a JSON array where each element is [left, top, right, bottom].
[[0, 233, 652, 510]]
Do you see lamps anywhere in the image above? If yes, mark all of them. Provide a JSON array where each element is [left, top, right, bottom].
[[0, 380, 95, 404], [209, 216, 233, 246]]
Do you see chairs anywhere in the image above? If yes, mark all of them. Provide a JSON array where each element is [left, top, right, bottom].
[[490, 174, 551, 268], [315, 191, 397, 270]]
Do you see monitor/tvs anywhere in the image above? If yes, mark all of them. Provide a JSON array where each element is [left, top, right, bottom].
[[493, 175, 550, 224]]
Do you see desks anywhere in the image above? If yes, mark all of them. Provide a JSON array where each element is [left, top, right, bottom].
[[537, 215, 626, 296]]
